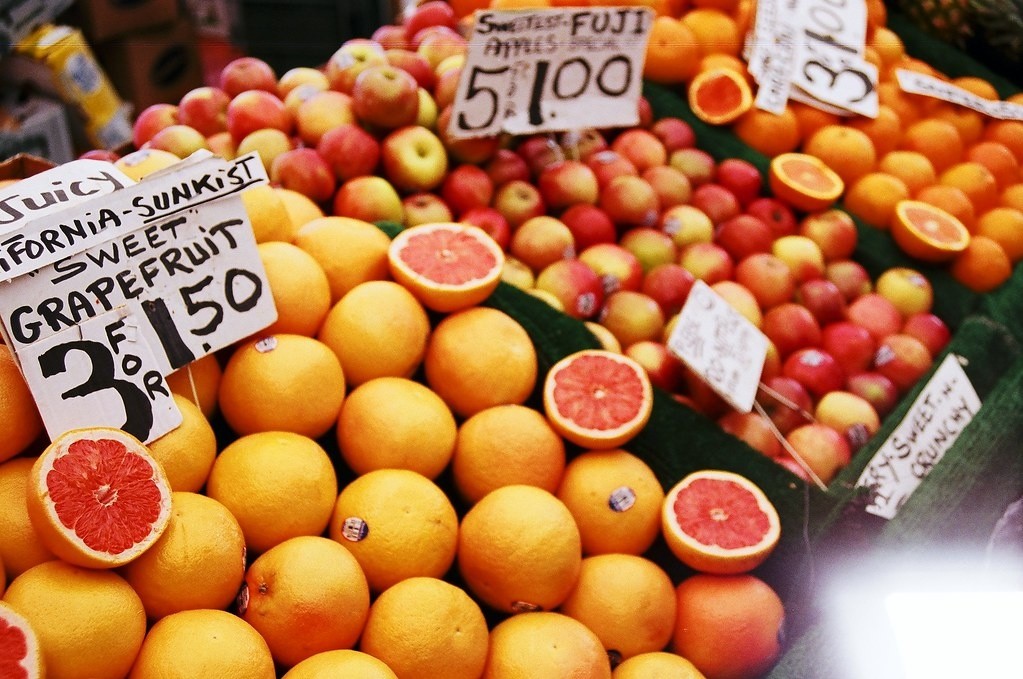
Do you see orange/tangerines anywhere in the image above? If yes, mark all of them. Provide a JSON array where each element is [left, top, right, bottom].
[[1, 145, 787, 679], [489, 0, 1022, 292]]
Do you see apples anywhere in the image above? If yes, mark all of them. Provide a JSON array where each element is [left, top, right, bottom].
[[85, 1, 949, 488]]
